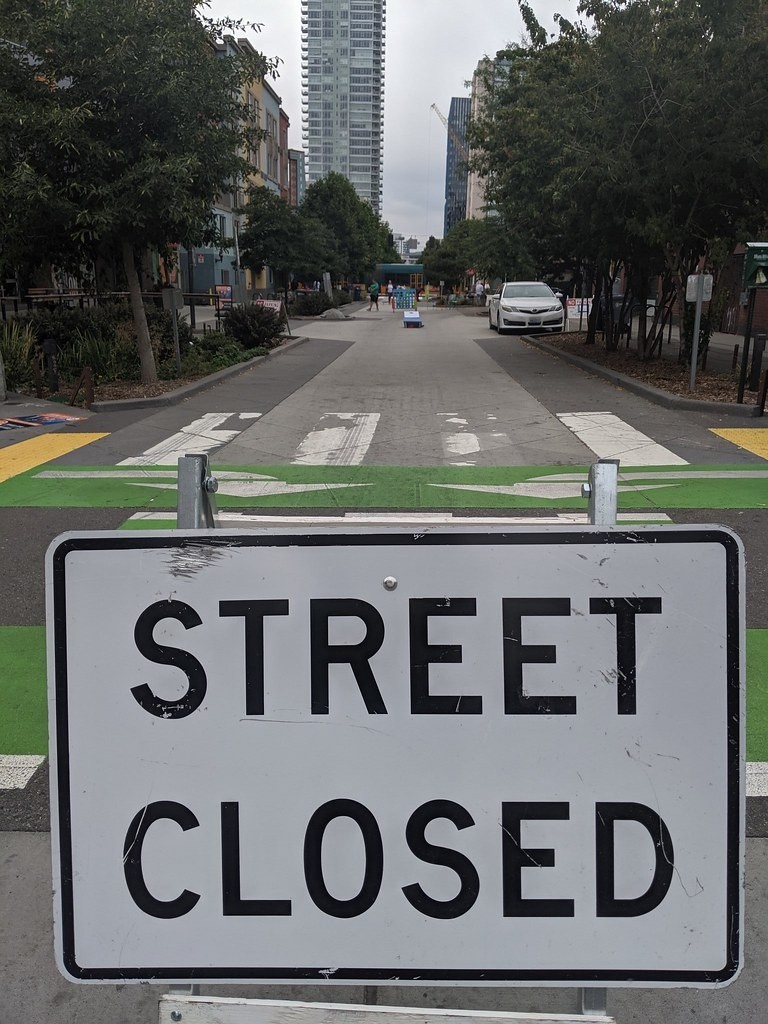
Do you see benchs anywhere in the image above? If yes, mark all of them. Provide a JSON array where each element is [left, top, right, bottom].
[[28, 287, 74, 313]]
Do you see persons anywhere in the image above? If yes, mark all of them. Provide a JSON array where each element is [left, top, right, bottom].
[[416, 288, 424, 302], [476, 282, 484, 298], [386, 279, 395, 304], [367, 279, 379, 311]]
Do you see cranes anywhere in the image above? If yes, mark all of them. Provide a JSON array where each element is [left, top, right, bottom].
[[430, 102, 468, 164]]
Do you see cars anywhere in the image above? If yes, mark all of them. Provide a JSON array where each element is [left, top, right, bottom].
[[487, 281, 565, 335]]
